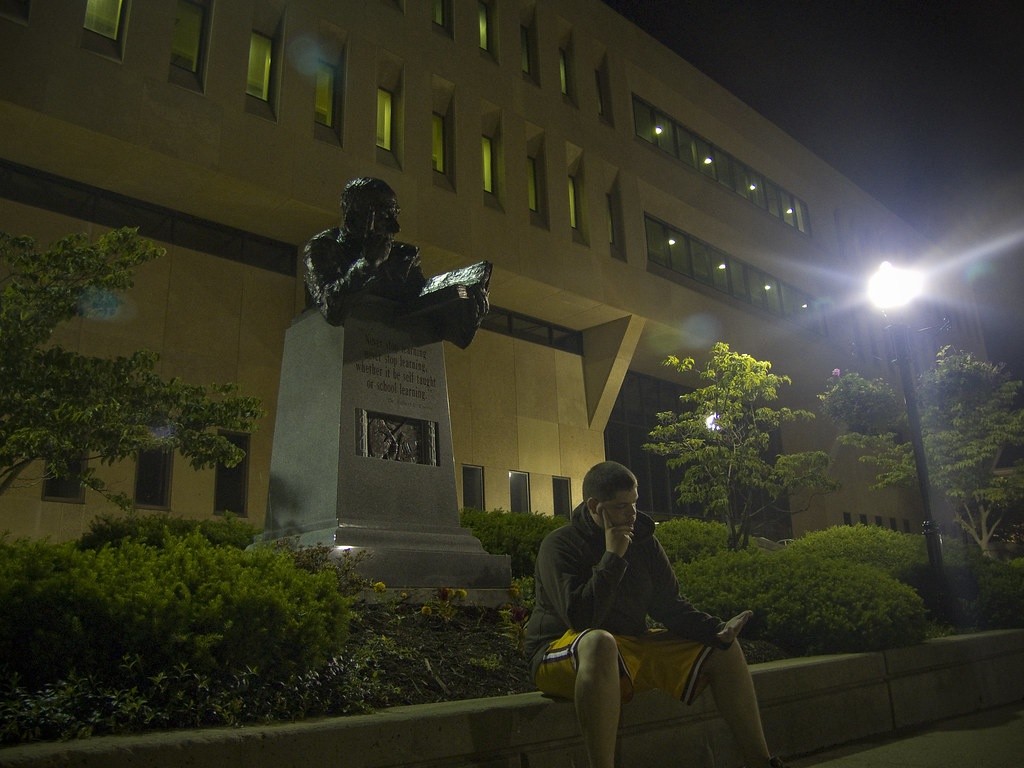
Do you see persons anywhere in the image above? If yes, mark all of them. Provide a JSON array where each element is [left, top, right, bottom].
[[304, 176, 493, 350], [523, 462, 787, 767]]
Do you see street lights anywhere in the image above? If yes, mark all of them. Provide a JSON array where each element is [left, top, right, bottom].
[[866, 261, 949, 623], [704, 412, 744, 551]]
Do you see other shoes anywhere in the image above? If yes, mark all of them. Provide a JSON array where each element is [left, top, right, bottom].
[[768, 756, 784, 768]]
